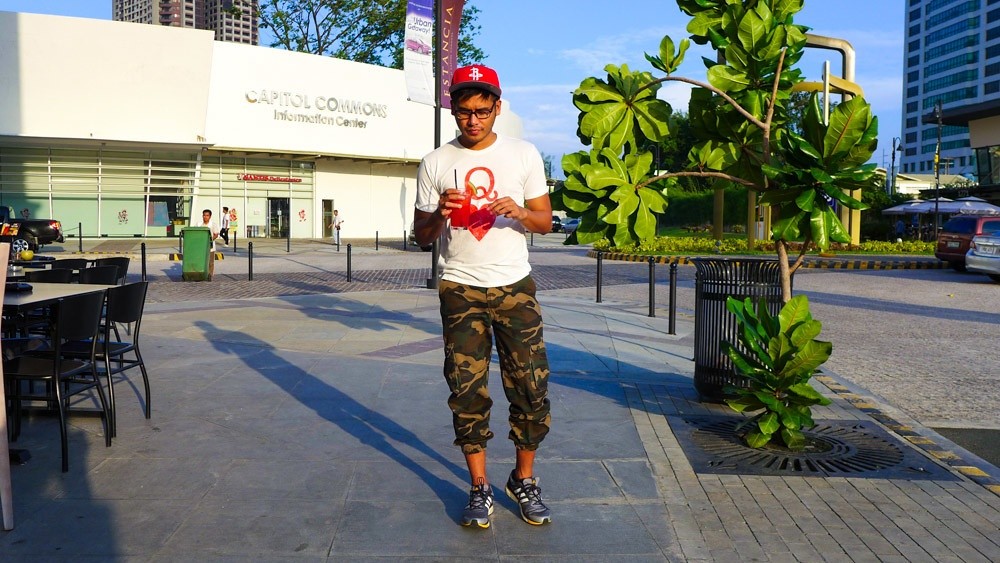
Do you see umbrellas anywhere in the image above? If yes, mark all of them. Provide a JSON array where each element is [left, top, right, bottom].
[[881, 195, 1000, 240]]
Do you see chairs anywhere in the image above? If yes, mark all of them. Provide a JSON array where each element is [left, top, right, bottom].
[[1, 257, 151, 474]]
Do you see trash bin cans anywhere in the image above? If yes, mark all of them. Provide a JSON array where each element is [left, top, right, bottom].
[[689, 257, 810, 405], [179, 226, 213, 281]]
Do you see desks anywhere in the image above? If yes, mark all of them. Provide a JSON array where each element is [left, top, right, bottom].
[[6, 268, 79, 277], [3, 282, 121, 417]]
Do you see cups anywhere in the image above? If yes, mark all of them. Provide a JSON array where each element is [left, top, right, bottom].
[[451, 189, 472, 227]]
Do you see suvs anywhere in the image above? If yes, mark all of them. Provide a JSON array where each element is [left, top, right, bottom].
[[934, 215, 1000, 275], [552, 215, 562, 233]]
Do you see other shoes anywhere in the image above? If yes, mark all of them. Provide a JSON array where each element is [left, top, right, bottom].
[[228, 238, 230, 244], [223, 245, 228, 248]]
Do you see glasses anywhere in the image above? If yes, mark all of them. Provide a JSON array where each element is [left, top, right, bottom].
[[454, 101, 496, 120]]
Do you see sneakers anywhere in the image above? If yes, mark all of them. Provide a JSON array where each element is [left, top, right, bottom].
[[460, 477, 494, 529], [505, 469, 552, 525]]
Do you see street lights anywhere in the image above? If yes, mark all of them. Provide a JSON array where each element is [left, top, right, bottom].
[[891, 136, 905, 196], [933, 97, 944, 241]]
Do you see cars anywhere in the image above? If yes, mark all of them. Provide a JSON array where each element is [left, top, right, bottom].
[[405, 37, 431, 55], [561, 219, 581, 233], [407, 219, 433, 252], [964, 230, 1000, 285]]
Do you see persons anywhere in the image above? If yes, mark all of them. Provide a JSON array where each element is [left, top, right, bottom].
[[220, 207, 230, 247], [414, 66, 552, 530], [196, 209, 219, 276], [894, 215, 904, 238], [905, 220, 912, 235], [928, 220, 934, 236], [328, 210, 344, 245]]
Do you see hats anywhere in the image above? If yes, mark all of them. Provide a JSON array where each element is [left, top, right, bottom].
[[449, 65, 502, 98]]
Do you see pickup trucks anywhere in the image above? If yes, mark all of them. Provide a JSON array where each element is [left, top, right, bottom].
[[0, 205, 66, 254]]
